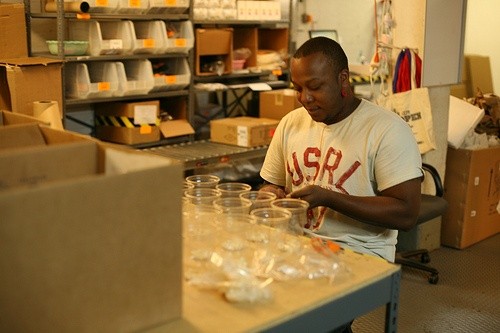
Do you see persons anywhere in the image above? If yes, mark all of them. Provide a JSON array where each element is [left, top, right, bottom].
[[258, 37, 426, 333]]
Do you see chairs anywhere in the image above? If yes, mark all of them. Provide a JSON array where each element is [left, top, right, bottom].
[[394, 163, 450, 286]]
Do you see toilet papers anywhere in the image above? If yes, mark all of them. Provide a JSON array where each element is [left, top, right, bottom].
[[45, 1, 90, 13]]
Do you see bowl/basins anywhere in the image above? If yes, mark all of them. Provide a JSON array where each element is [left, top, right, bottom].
[[233, 60, 247, 70], [46, 40, 90, 56]]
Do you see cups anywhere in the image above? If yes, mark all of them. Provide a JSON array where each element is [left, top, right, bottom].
[[182, 174, 310, 303]]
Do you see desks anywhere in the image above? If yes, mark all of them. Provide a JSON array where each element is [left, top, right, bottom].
[[130, 193, 404, 333]]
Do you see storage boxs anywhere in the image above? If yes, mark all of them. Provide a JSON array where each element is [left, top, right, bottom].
[[231, 26, 290, 69], [440, 142, 500, 249], [93, 119, 161, 145], [260, 89, 304, 120], [90, 100, 160, 118], [159, 96, 196, 139], [0, 109, 185, 333], [209, 117, 281, 148], [194, 27, 233, 75]]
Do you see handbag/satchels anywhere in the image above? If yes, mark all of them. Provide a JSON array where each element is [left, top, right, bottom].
[[375, 46, 439, 155]]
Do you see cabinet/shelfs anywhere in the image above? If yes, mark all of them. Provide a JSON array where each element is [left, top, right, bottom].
[[23, 0, 296, 191]]
